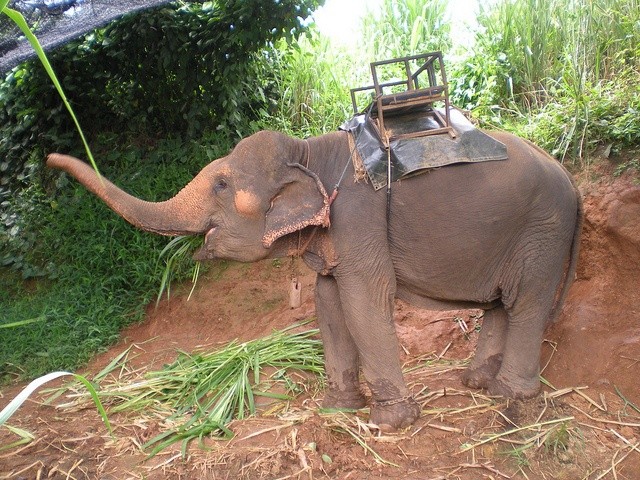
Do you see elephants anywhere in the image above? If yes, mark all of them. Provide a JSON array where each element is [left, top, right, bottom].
[[45, 105, 584, 435]]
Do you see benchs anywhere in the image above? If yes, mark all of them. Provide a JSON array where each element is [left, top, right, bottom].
[[348, 50, 458, 150]]
